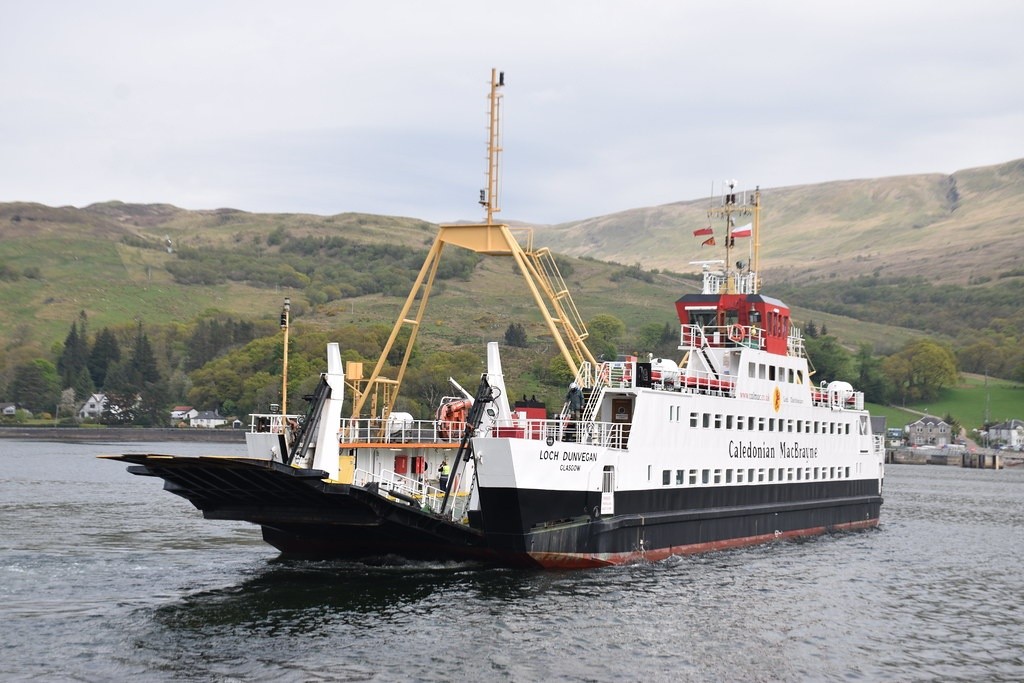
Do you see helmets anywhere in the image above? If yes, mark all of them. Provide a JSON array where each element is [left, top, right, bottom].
[[569, 383, 576, 388]]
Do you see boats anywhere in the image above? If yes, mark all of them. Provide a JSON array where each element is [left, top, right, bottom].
[[434, 397, 473, 443], [95, 66, 886, 571]]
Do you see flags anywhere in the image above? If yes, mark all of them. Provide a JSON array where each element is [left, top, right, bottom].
[[694, 228, 712, 236], [731, 222, 752, 237], [702, 237, 715, 246]]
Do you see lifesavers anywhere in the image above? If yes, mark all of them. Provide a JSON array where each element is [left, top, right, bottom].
[[728, 324, 745, 342]]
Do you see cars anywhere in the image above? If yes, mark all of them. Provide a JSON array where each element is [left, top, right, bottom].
[[958, 439, 966, 446], [990, 442, 1024, 451]]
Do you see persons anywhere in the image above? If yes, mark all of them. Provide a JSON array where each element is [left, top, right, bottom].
[[438, 459, 450, 493], [565, 382, 584, 420]]
